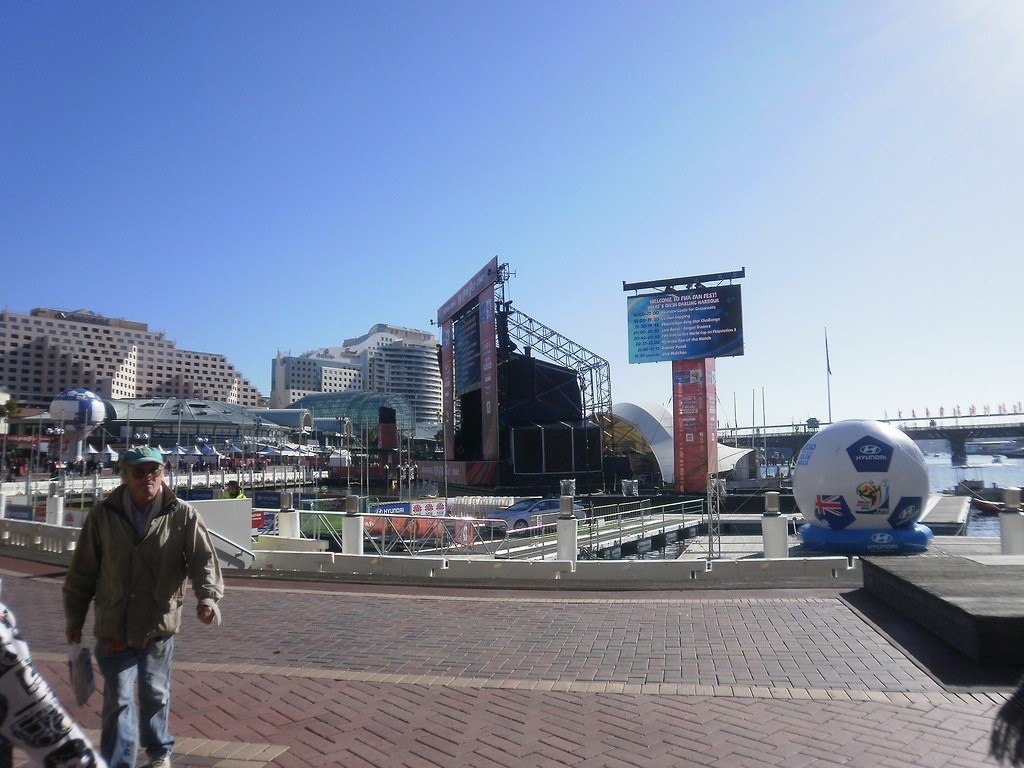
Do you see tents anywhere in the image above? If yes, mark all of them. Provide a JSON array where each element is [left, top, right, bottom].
[[254, 446, 282, 466], [81, 442, 222, 464]]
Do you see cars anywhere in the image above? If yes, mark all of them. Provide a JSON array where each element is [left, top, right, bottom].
[[485, 499, 588, 536]]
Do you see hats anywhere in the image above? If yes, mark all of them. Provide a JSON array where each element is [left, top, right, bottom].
[[123, 446, 164, 466]]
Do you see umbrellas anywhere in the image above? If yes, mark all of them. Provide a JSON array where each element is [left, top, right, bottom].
[[219, 443, 243, 458]]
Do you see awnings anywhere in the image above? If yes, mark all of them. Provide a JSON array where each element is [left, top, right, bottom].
[[277, 448, 319, 458]]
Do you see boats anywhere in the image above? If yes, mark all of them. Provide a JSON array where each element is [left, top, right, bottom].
[[992, 454, 1008, 465], [972, 497, 1024, 516]]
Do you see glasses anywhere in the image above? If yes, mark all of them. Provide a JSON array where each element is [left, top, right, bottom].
[[131, 467, 163, 479]]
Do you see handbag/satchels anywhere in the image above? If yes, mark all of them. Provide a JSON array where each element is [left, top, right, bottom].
[[67, 635, 96, 708]]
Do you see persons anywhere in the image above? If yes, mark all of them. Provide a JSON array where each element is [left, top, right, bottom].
[[222, 481, 246, 499], [61, 446, 224, 768], [5, 449, 269, 483], [0, 577, 109, 768]]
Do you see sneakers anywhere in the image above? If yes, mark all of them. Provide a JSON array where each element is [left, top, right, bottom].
[[148, 754, 170, 768]]
